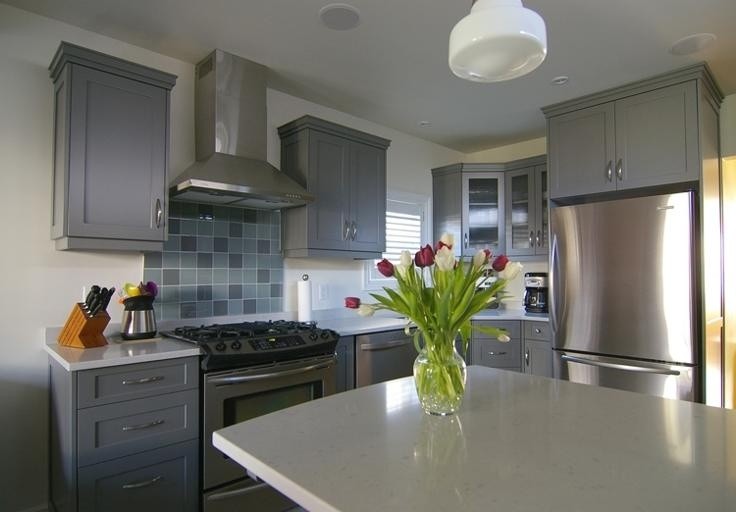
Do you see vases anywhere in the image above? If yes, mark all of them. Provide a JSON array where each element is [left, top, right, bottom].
[[413, 339, 468, 418]]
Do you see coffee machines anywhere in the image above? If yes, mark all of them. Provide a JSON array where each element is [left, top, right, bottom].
[[473, 266, 500, 309], [522, 273, 549, 313]]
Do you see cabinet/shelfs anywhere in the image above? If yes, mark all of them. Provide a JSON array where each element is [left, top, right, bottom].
[[276, 113, 393, 264], [538, 61, 725, 207], [354, 319, 553, 387], [42, 351, 201, 512], [422, 161, 506, 266], [46, 39, 179, 253], [506, 153, 550, 263]]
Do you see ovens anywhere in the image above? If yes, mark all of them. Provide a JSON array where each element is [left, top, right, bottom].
[[200, 354, 336, 512]]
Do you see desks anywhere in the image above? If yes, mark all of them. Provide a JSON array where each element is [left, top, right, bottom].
[[209, 361, 736, 512]]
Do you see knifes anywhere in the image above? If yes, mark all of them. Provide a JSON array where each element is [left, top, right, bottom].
[[81, 285, 114, 319]]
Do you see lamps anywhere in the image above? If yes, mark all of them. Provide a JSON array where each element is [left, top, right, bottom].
[[445, 1, 550, 84]]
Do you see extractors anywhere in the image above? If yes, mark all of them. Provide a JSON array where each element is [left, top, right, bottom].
[[166, 48, 316, 215]]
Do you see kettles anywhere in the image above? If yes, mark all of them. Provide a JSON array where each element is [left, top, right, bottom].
[[122, 293, 159, 339]]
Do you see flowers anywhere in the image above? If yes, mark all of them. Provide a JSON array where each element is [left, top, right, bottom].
[[342, 232, 531, 398]]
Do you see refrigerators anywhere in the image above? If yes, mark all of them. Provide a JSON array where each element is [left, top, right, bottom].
[[543, 191, 702, 403]]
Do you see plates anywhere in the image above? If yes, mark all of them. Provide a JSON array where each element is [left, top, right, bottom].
[[476, 207, 548, 220], [468, 190, 493, 200], [510, 189, 549, 199]]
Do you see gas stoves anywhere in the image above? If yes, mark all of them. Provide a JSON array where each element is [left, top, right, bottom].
[[171, 319, 343, 368]]
